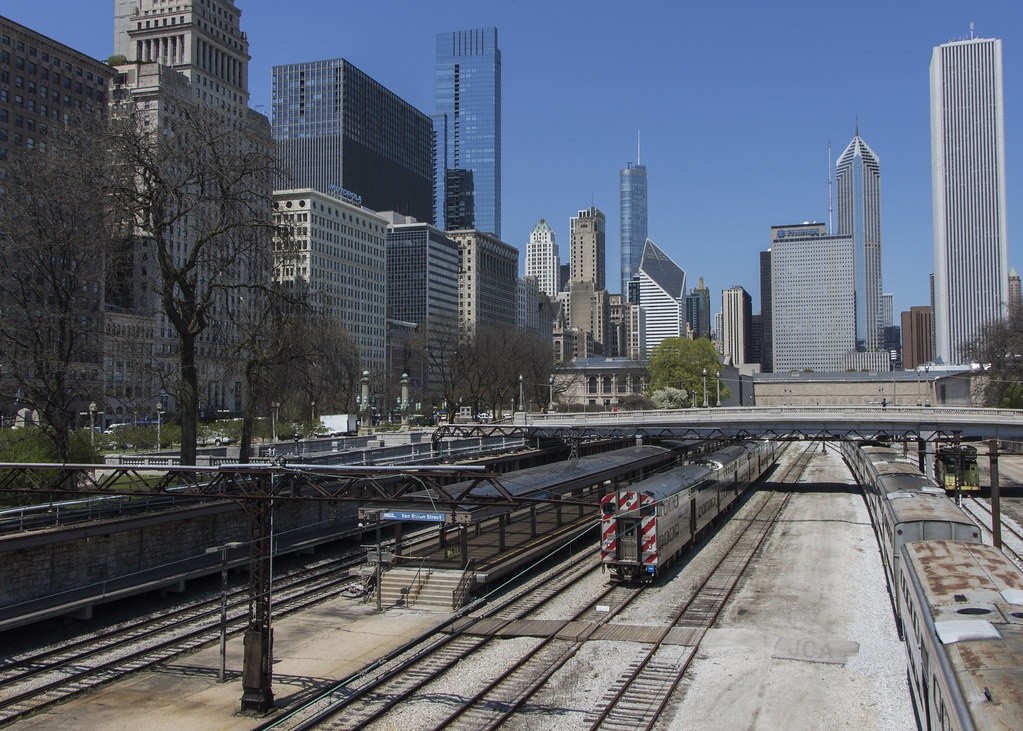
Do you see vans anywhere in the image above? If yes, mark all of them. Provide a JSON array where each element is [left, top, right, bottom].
[[103, 423, 133, 441]]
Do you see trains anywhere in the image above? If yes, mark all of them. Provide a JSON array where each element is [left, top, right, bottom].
[[840, 438, 1023, 731], [596, 440, 779, 585]]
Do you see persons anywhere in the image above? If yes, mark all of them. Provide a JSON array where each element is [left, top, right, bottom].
[[880, 398, 889, 410], [613, 405, 618, 416], [541, 407, 549, 418], [372, 417, 380, 426]]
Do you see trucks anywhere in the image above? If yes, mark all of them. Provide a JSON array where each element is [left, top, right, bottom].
[[314, 413, 357, 438]]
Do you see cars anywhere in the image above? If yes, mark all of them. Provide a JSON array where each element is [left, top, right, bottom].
[[196, 429, 236, 447]]
